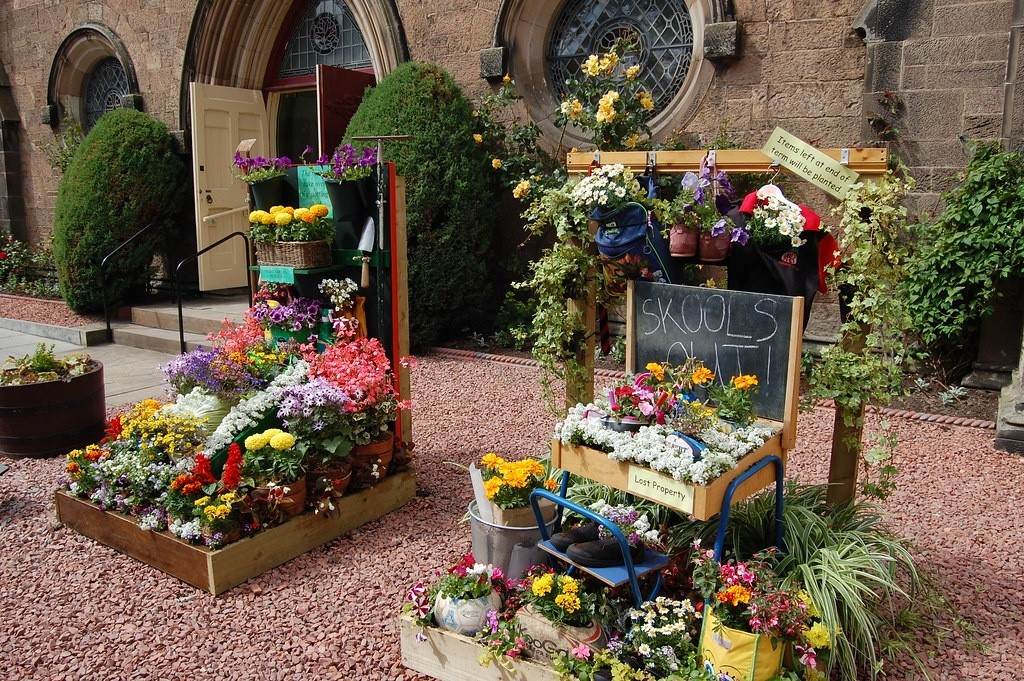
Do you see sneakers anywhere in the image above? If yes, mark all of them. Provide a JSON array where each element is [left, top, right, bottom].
[[549, 523, 601, 551], [670, 222, 699, 257], [566, 538, 644, 565], [699, 229, 733, 261]]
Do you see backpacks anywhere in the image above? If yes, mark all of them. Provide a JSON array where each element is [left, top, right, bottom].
[[591, 202, 682, 296]]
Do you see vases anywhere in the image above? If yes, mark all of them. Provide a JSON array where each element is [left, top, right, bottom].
[[697, 227, 731, 258], [351, 429, 396, 480], [323, 175, 374, 219], [254, 473, 306, 519], [501, 605, 600, 665], [668, 223, 691, 255], [251, 237, 321, 267], [265, 319, 314, 349], [485, 494, 555, 527], [432, 582, 501, 634], [247, 174, 288, 209], [309, 457, 351, 498]]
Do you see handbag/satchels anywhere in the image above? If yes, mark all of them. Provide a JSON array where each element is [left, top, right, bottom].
[[696, 601, 787, 681]]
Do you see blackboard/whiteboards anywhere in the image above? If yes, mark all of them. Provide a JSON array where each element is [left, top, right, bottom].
[[626, 281, 805, 422]]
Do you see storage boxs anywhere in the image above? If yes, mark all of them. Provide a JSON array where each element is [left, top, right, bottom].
[[552, 430, 787, 521]]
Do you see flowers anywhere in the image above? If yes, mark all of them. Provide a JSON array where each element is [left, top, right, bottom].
[[409, 45, 923, 681], [61, 137, 414, 552]]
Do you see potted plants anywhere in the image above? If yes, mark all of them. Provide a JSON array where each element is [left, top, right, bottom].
[[1, 343, 107, 457]]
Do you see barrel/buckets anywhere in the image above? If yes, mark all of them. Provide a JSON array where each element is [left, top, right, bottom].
[[469, 499, 559, 580]]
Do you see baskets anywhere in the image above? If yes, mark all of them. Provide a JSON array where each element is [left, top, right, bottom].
[[256, 239, 332, 269]]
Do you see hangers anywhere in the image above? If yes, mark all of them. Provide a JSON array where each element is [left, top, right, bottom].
[[755, 162, 799, 211]]
